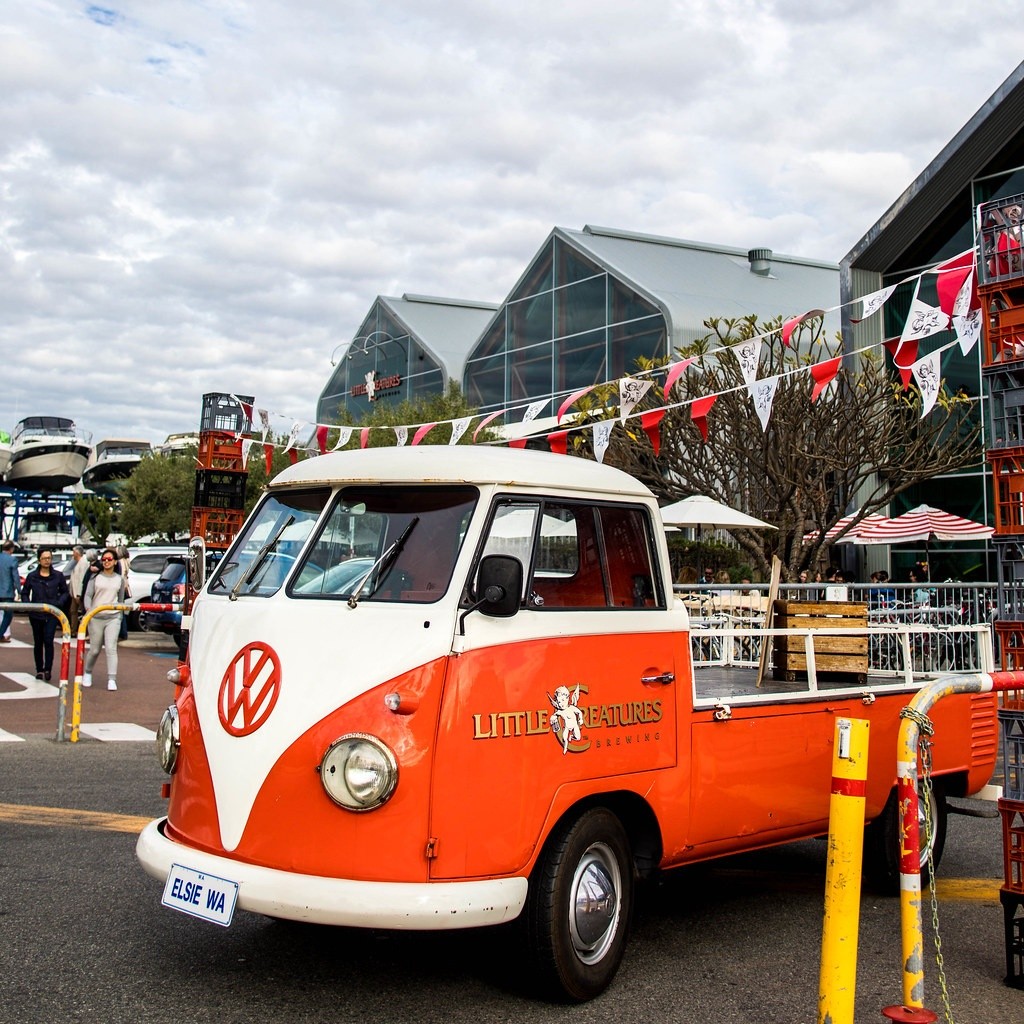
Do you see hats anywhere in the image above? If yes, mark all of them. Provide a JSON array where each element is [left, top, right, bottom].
[[826, 567, 837, 578]]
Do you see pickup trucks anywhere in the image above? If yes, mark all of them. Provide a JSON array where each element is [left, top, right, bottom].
[[134, 443, 999, 1008]]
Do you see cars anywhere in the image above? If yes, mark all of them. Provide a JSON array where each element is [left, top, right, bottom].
[[10, 549, 75, 601]]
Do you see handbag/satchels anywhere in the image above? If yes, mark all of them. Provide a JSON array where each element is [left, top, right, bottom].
[[124, 585, 131, 600]]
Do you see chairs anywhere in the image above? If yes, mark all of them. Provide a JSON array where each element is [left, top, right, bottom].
[[381, 569, 412, 596]]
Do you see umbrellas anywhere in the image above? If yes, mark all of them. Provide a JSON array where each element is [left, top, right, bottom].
[[658, 493, 780, 583], [801, 502, 997, 583]]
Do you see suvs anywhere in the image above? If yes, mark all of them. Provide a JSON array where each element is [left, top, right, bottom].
[[296, 557, 375, 597], [149, 549, 323, 648], [121, 546, 218, 632]]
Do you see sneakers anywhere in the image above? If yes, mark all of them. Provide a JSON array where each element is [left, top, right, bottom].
[[107, 680, 118, 690], [83, 672, 92, 687]]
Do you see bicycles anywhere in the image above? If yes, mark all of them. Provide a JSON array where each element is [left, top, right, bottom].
[[682, 589, 997, 673]]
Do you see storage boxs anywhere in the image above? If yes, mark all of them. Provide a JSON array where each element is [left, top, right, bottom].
[[772, 596, 872, 682]]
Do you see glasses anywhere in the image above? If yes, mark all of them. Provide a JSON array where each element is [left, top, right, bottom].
[[102, 557, 113, 561], [705, 572, 713, 575]]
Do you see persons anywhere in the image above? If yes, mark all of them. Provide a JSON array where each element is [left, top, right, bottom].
[[69, 545, 133, 691], [0, 540, 22, 644], [20, 546, 68, 681], [676, 565, 762, 598], [800, 566, 936, 605]]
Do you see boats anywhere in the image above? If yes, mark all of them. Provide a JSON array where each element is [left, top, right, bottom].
[[82, 438, 156, 496], [154, 431, 202, 457], [0, 415, 93, 492]]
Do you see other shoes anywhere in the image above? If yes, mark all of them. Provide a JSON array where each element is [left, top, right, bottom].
[[0, 636, 12, 642], [36, 672, 43, 680], [45, 670, 52, 681]]
